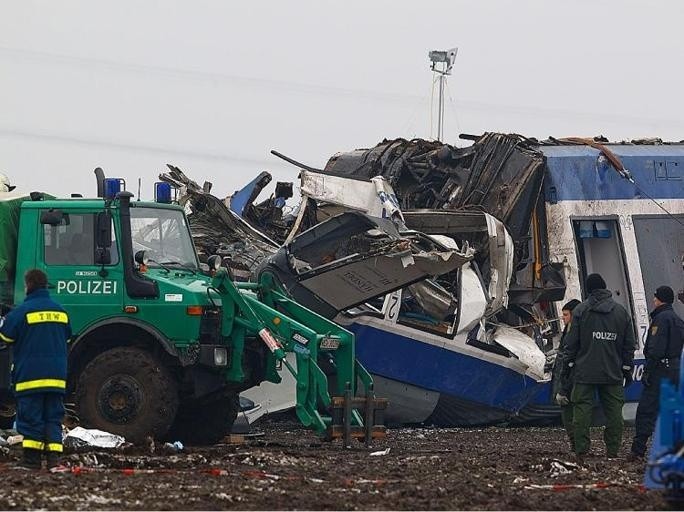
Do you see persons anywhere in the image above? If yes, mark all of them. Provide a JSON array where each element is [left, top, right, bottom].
[[564, 273, 635, 458], [626, 285, 684, 463], [1, 270, 74, 472], [552, 299, 581, 452]]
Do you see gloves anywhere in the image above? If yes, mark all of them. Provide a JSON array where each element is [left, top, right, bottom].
[[555, 394, 570, 407], [642, 374, 651, 387], [623, 370, 634, 388]]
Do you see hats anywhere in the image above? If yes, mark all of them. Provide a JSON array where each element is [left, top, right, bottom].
[[653, 285, 674, 303], [587, 273, 606, 292]]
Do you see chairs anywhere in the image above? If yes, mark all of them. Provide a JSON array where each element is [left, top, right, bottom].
[[94, 167, 126, 196], [153, 181, 178, 203]]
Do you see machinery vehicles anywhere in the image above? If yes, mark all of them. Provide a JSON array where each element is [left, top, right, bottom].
[[0, 177, 392, 449]]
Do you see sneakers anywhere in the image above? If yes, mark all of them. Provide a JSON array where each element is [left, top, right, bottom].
[[48, 453, 59, 468], [16, 458, 42, 470], [626, 452, 642, 462]]
